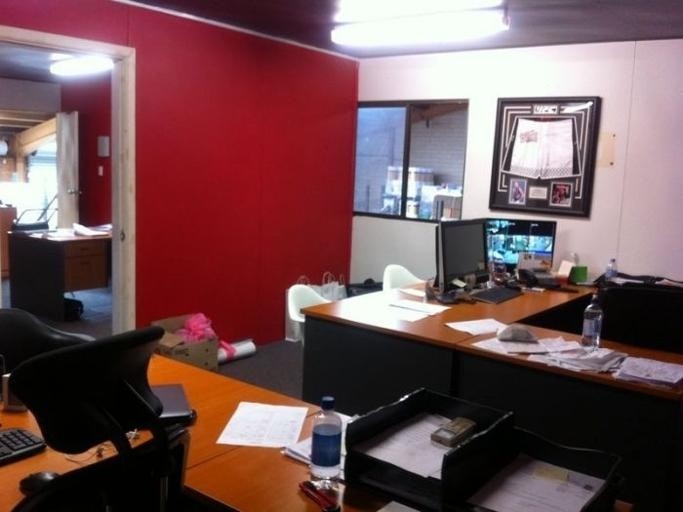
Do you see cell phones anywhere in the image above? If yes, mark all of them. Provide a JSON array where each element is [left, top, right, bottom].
[[432, 415, 477, 445], [435, 294, 456, 304]]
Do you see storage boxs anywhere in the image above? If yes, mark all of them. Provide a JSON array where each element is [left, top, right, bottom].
[[149, 313, 219, 373]]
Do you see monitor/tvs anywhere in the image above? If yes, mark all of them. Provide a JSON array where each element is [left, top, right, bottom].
[[433, 218, 490, 309], [487, 217, 560, 289]]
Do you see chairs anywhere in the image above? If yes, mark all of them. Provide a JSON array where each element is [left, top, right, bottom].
[[8, 326, 191, 512], [12, 221, 75, 298], [382, 263, 427, 289], [286, 283, 332, 346]]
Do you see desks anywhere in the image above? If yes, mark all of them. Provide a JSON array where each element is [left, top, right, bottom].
[[456, 320, 682, 511], [300, 279, 594, 416], [0, 353, 323, 512], [181, 411, 634, 512]]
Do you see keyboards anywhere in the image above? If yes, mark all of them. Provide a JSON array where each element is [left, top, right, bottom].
[[475, 285, 524, 304], [0, 428, 47, 468]]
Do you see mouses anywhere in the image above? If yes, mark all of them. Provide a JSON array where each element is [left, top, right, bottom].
[[497, 326, 539, 343]]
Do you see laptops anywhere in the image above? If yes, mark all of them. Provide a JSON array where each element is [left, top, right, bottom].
[[147, 383, 198, 428]]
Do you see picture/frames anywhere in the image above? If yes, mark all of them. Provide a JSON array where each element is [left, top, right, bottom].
[[488, 96, 602, 219]]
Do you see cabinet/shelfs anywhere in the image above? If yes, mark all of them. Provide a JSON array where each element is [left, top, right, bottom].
[[7, 227, 112, 293]]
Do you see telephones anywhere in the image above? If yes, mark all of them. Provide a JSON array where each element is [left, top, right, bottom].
[[522, 268, 558, 286]]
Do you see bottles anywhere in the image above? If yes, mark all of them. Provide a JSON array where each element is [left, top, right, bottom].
[[310, 397, 342, 490], [605, 258, 617, 282], [581, 293, 604, 346]]
